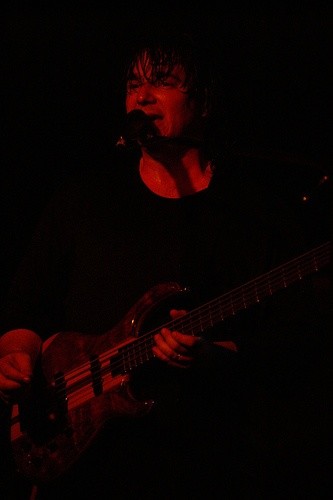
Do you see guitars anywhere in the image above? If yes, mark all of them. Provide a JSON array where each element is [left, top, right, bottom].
[[9, 241, 333, 485]]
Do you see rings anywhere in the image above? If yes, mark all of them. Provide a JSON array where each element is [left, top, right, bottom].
[[173, 352, 181, 361]]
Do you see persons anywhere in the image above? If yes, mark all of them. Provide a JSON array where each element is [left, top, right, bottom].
[[1, 29, 309, 498]]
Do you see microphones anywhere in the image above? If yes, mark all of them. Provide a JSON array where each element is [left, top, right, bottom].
[[113, 109, 151, 165]]
